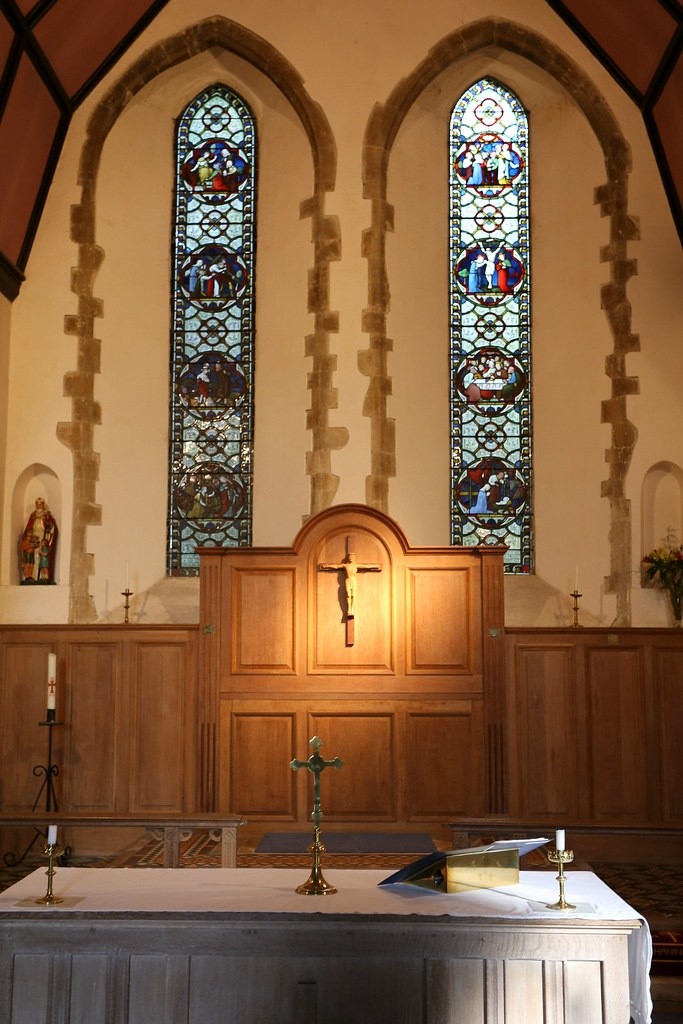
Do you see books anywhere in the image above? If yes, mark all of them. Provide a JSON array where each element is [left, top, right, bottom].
[[376, 836, 554, 886]]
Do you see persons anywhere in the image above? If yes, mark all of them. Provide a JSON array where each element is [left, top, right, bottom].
[[323, 553, 379, 616], [20, 497, 58, 584]]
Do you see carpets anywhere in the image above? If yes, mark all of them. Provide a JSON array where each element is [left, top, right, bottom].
[[255, 832, 437, 854]]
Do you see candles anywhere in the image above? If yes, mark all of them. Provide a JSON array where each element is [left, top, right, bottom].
[[47, 653, 56, 710], [125, 562, 129, 590], [555, 829, 565, 850], [48, 825, 57, 844], [575, 565, 579, 591]]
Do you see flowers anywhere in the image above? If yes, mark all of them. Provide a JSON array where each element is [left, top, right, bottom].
[[640, 526, 683, 594]]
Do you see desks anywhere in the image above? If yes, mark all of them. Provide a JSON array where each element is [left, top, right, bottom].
[[1, 866, 643, 1024]]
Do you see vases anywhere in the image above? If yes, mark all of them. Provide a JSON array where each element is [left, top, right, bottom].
[[670, 589, 683, 628]]
